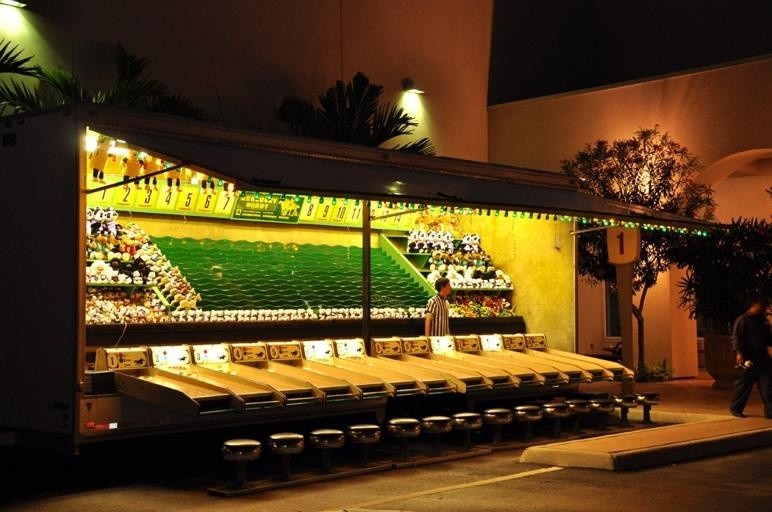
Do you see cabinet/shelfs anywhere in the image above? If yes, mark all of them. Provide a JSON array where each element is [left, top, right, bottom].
[[380, 222, 519, 326], [85, 210, 209, 323]]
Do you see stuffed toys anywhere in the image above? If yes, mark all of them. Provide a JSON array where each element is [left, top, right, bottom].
[[406, 228, 516, 319], [85, 205, 204, 324], [86, 134, 240, 199], [204, 307, 406, 323]]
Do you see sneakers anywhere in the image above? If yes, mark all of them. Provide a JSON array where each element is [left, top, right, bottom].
[[733, 412, 745, 417]]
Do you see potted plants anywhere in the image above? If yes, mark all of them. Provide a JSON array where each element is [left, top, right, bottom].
[[664, 215, 772, 391]]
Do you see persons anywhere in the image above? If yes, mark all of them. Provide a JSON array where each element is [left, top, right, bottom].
[[424, 277, 452, 336], [728, 294, 771, 419]]
